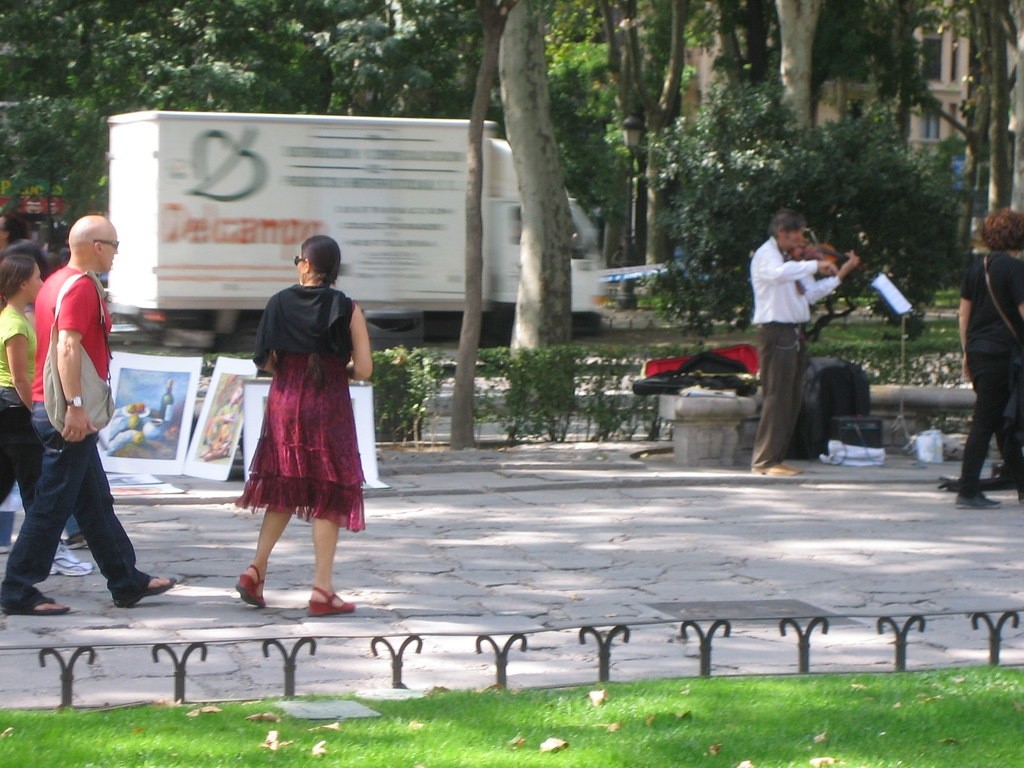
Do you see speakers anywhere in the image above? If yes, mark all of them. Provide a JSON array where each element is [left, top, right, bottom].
[[829, 413, 884, 449]]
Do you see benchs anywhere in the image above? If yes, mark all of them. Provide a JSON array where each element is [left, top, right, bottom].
[[660, 385, 979, 466]]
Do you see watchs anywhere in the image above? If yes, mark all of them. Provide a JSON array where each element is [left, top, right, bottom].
[[66, 397, 84, 407]]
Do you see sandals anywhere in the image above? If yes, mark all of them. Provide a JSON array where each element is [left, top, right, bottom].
[[114, 577, 177, 606], [0, 596, 69, 616]]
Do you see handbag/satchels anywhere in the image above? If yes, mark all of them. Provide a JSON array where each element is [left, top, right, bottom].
[[41, 274, 116, 432]]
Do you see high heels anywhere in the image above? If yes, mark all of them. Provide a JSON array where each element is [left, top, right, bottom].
[[308, 587, 355, 616], [236, 565, 266, 607]]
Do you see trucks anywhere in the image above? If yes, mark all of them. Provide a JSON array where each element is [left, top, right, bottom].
[[106, 112, 609, 355]]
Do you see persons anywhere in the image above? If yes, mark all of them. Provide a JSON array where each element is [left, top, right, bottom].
[[0, 210, 176, 616], [953, 208, 1024, 509], [750, 206, 863, 476], [233, 233, 373, 618]]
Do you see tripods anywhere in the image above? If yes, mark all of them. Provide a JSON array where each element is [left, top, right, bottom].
[[872, 275, 919, 459]]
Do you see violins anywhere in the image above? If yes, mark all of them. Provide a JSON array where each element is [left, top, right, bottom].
[[791, 243, 868, 271]]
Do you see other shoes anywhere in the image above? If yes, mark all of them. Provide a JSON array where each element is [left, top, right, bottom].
[[752, 463, 802, 477], [956, 494, 1001, 509], [65, 535, 88, 550]]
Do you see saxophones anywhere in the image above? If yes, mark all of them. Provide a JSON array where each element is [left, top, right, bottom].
[[673, 366, 763, 386]]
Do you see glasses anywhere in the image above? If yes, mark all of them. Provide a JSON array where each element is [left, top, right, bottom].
[[294, 256, 312, 266], [94, 240, 120, 251]]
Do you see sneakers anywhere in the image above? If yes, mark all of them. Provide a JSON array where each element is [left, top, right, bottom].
[[49, 544, 95, 576]]
